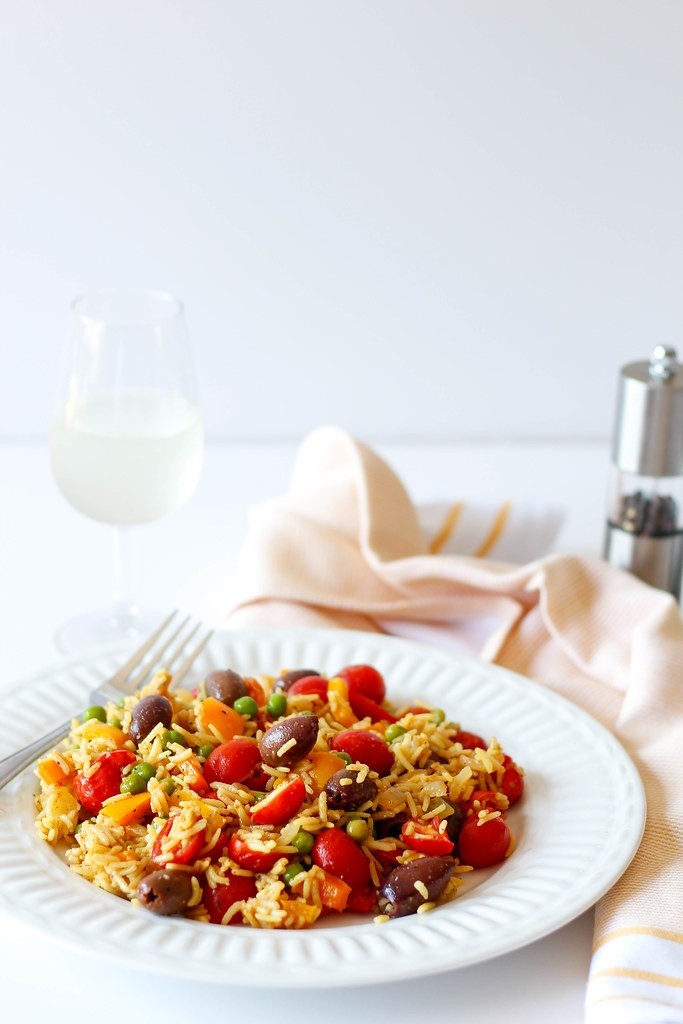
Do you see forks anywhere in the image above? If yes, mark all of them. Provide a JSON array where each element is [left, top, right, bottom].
[[0, 608, 214, 795]]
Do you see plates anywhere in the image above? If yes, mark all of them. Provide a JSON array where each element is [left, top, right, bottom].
[[0, 628, 646, 995]]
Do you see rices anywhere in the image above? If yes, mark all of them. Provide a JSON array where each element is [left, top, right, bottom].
[[32, 666, 524, 928]]
